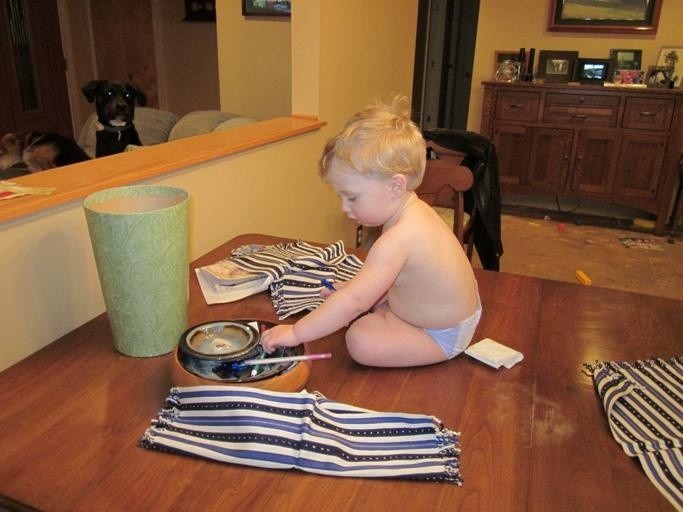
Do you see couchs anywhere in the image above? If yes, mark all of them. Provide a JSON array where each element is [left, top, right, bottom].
[[77, 108, 260, 158]]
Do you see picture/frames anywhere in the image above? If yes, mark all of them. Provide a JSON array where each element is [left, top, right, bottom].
[[537, 51, 579, 82], [241, 0, 292, 16], [546, 0, 663, 34], [576, 58, 613, 85], [656, 46, 683, 87], [495, 50, 529, 74], [610, 49, 643, 70]]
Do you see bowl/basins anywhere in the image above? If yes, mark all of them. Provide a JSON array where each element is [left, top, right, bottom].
[[170, 318, 315, 400]]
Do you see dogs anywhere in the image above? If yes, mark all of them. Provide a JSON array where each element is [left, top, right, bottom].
[[81, 79, 148, 159], [0, 130, 90, 173]]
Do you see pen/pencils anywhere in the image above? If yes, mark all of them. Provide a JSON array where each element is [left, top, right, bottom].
[[320, 278, 336, 293], [244, 353, 332, 366], [251, 350, 266, 377]]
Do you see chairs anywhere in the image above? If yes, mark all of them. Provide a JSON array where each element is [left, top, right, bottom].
[[413, 129, 476, 264]]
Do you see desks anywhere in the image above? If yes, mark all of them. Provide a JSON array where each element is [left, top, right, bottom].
[[1, 233, 683, 511]]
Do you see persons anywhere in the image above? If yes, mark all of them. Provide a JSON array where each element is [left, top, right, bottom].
[[258, 94, 482, 367]]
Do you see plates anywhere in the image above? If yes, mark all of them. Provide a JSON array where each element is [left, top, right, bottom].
[[181, 319, 258, 362]]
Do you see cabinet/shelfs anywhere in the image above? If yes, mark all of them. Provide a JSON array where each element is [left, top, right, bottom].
[[478, 82, 683, 236]]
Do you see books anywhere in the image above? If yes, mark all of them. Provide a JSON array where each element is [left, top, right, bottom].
[[199, 261, 266, 294]]
